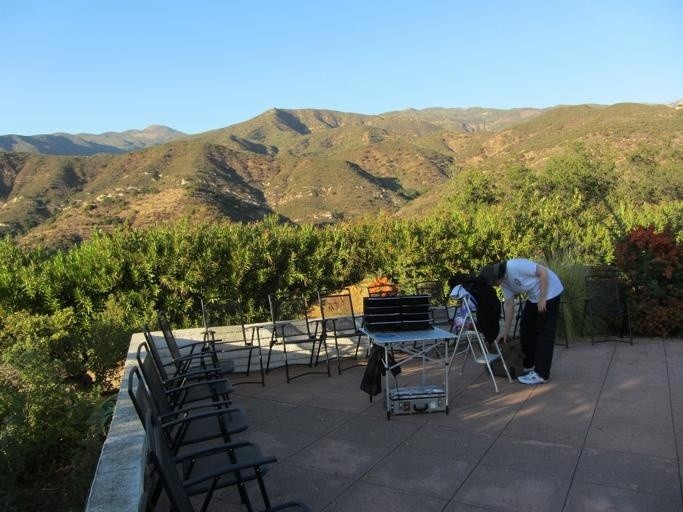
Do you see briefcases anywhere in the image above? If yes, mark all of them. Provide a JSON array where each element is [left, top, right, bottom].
[[384, 385, 446, 415]]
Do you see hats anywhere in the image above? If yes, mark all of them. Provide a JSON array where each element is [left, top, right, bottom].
[[479, 265, 497, 286]]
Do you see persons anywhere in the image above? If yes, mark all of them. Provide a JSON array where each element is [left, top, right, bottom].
[[476, 258, 564, 385]]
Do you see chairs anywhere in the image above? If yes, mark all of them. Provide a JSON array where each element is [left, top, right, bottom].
[[199, 281, 462, 387], [130, 307, 276, 512], [583, 271, 635, 351]]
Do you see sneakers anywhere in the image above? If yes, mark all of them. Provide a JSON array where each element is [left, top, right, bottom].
[[517, 364, 551, 384]]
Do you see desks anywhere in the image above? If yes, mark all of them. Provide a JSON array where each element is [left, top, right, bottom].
[[360, 323, 460, 415]]
